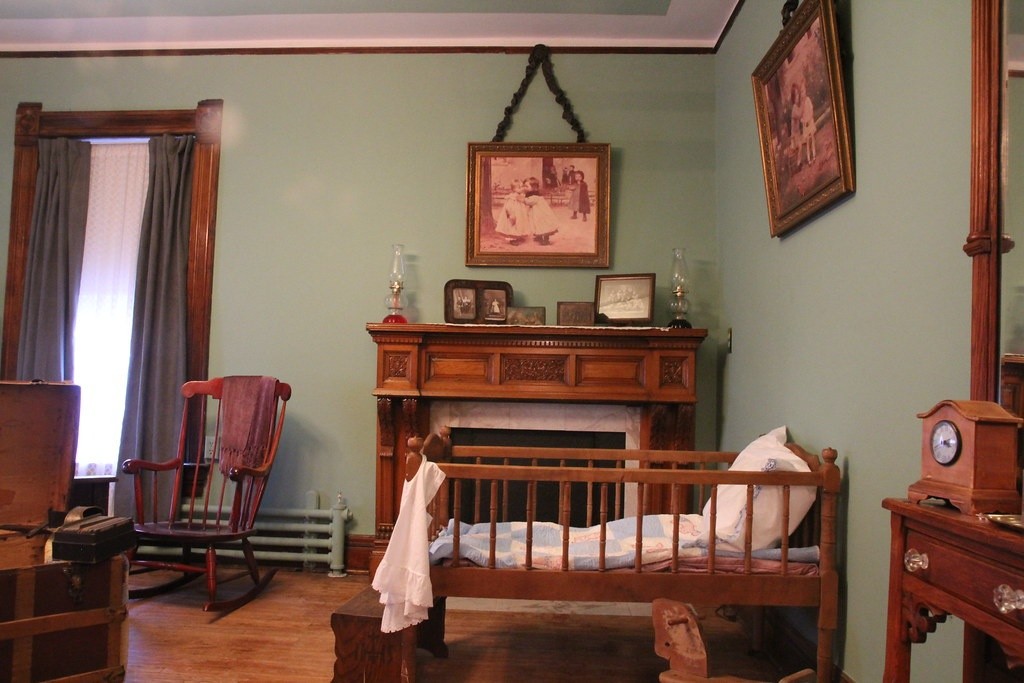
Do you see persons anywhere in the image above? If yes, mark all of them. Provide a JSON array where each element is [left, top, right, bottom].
[[547, 165, 576, 190], [457, 295, 472, 313], [568, 171, 591, 222], [490, 298, 500, 315], [495, 177, 559, 246], [790, 79, 817, 172]]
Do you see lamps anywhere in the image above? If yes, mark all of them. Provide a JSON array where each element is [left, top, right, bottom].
[[666, 246, 692, 327], [383, 244, 411, 324]]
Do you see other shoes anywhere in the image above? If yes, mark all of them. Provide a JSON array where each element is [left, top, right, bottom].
[[517, 238, 523, 242], [583, 217, 587, 220], [510, 240, 518, 246], [534, 236, 541, 240], [571, 214, 576, 219], [799, 163, 802, 171], [808, 161, 812, 167], [544, 240, 549, 244], [814, 159, 816, 162]]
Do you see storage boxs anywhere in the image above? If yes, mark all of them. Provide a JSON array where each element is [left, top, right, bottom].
[[0, 379, 130, 683]]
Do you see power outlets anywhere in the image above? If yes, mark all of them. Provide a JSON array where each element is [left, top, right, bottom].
[[204, 436, 220, 460]]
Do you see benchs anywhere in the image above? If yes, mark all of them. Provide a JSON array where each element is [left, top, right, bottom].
[[330, 582, 448, 683]]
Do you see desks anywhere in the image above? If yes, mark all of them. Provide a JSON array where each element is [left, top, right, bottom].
[[69, 476, 120, 516], [881, 498, 1024, 683], [362, 321, 709, 581]]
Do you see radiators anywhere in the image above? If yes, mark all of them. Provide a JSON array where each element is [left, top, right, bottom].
[[139, 489, 352, 578]]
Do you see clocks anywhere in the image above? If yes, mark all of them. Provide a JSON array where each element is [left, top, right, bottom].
[[930, 418, 962, 466]]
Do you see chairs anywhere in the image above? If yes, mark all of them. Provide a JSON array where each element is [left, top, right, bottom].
[[122, 375, 291, 612]]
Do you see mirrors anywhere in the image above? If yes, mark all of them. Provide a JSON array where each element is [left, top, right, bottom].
[[969, 0, 1024, 494]]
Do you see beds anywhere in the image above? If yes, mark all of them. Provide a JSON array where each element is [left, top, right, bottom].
[[398, 424, 842, 683]]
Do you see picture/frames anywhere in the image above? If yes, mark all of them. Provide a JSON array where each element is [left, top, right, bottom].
[[464, 142, 611, 268], [442, 278, 514, 324], [593, 272, 656, 323], [557, 301, 595, 326], [505, 307, 546, 326], [748, 0, 856, 238]]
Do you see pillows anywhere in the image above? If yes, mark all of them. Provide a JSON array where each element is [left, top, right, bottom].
[[701, 424, 816, 553]]
